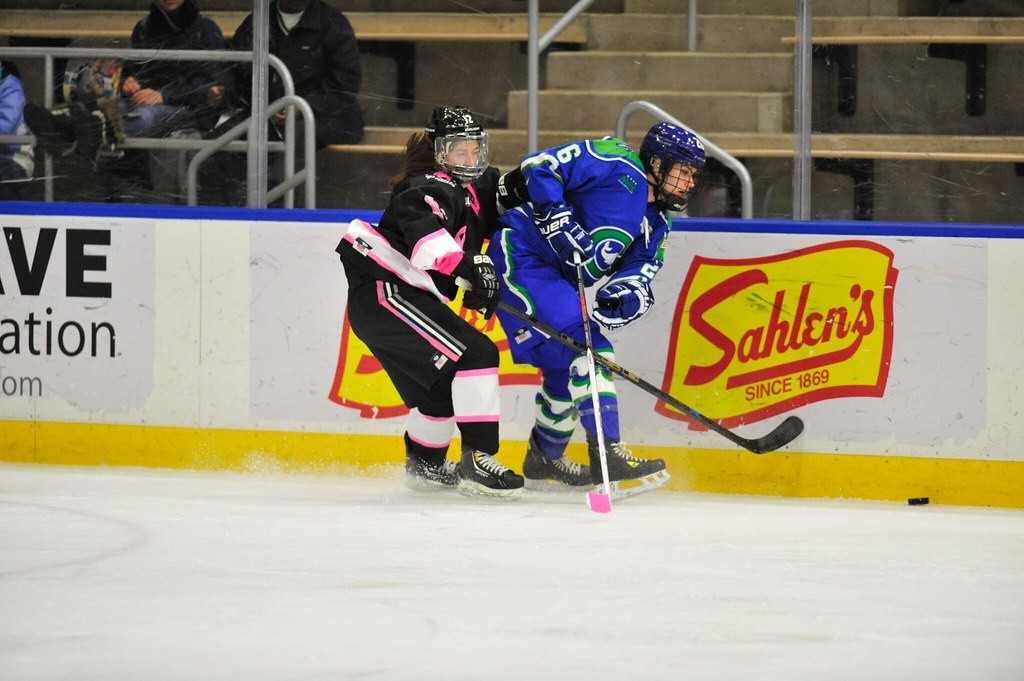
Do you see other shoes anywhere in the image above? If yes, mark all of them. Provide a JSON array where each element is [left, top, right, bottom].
[[23, 102, 115, 175]]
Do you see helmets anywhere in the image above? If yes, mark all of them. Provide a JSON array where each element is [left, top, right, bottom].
[[424, 105, 490, 182], [638, 122, 707, 212]]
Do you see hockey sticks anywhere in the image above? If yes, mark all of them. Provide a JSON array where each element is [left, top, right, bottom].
[[493, 296, 805, 454], [572, 245, 611, 512]]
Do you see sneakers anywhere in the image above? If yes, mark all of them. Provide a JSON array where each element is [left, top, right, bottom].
[[456, 449, 524, 502], [523, 428, 595, 492], [405, 456, 470, 500], [585, 433, 671, 511]]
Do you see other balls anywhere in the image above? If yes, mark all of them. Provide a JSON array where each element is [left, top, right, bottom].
[[908, 497, 930, 504]]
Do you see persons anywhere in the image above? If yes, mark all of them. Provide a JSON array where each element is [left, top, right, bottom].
[[484, 123, 706, 501], [347, 106, 526, 503], [184, 0, 366, 207], [0, 57, 37, 200], [23, 0, 226, 178]]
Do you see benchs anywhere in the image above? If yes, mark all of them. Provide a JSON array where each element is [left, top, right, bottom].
[[0, 0, 1024, 163]]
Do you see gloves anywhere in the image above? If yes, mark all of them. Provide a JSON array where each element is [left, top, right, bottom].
[[530, 207, 596, 268], [589, 279, 652, 330], [454, 249, 503, 320]]
